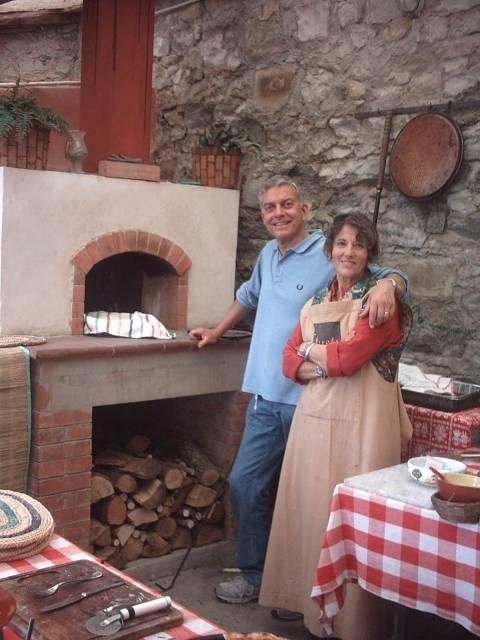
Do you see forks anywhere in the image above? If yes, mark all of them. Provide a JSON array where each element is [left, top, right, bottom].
[[32, 570, 102, 600]]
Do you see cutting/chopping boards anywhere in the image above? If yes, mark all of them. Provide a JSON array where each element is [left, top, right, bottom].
[[0, 559, 183, 640], [344, 462, 439, 511]]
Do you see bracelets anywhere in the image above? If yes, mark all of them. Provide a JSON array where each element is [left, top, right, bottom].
[[376, 278, 398, 288], [298, 341, 315, 360], [315, 365, 326, 380]]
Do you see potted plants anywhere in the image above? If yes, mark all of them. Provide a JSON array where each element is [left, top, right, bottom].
[[191, 120, 265, 189], [0, 77, 73, 170]]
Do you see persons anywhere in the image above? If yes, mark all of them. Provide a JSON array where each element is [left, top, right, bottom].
[[256, 212, 415, 639], [188, 176, 409, 618]]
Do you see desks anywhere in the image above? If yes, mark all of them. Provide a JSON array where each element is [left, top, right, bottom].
[[311, 445, 480, 640], [0, 532, 231, 640], [402, 405, 480, 461]]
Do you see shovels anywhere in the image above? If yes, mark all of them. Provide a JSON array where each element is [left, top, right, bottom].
[[86, 596, 172, 637]]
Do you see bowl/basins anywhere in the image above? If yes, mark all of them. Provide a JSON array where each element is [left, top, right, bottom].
[[407, 455, 466, 488], [431, 495, 480, 524], [434, 473, 480, 500]]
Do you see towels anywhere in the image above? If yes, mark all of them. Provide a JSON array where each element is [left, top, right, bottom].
[[85, 309, 176, 340], [399, 362, 454, 395]]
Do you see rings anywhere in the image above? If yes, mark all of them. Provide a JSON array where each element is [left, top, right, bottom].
[[384, 311, 389, 314]]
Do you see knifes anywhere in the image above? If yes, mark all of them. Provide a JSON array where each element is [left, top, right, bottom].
[[39, 578, 126, 614]]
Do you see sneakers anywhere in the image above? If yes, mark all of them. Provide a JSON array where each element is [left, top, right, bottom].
[[215, 576, 260, 604], [271, 606, 304, 620]]
